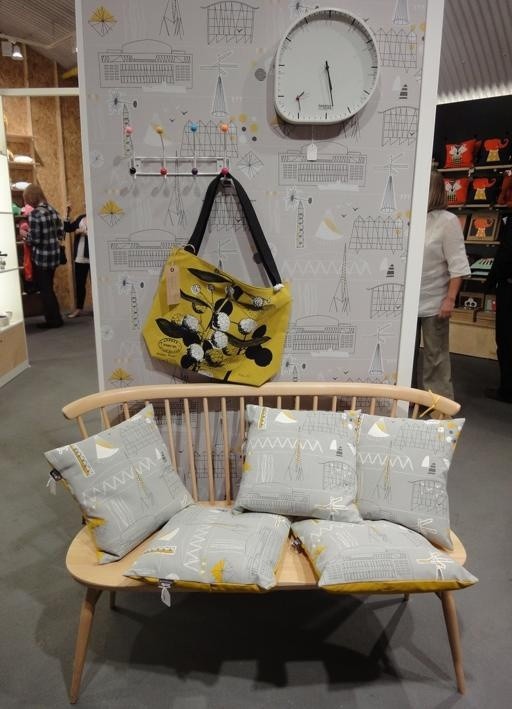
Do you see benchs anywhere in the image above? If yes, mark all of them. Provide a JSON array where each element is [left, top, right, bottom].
[[61, 379, 469, 704]]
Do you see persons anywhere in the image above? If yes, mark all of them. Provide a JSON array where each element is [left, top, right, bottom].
[[408, 171, 473, 421], [474, 208, 512, 404], [21, 182, 66, 328], [62, 201, 90, 319]]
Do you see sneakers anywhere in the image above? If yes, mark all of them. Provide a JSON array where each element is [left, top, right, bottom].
[[485, 387, 511, 403], [67, 309, 81, 318]]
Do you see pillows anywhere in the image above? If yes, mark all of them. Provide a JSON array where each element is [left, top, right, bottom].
[[123, 505, 292, 594], [290, 520, 479, 594], [231, 404, 465, 552], [43, 403, 196, 565]]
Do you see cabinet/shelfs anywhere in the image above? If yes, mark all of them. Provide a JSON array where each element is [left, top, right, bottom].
[[5, 132, 43, 296], [419, 164, 512, 359]]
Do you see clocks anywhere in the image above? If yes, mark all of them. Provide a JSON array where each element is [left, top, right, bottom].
[[273, 8, 383, 129]]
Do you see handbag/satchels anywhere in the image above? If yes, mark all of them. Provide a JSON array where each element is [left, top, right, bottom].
[[140, 245, 289, 387], [58, 245, 67, 265]]
